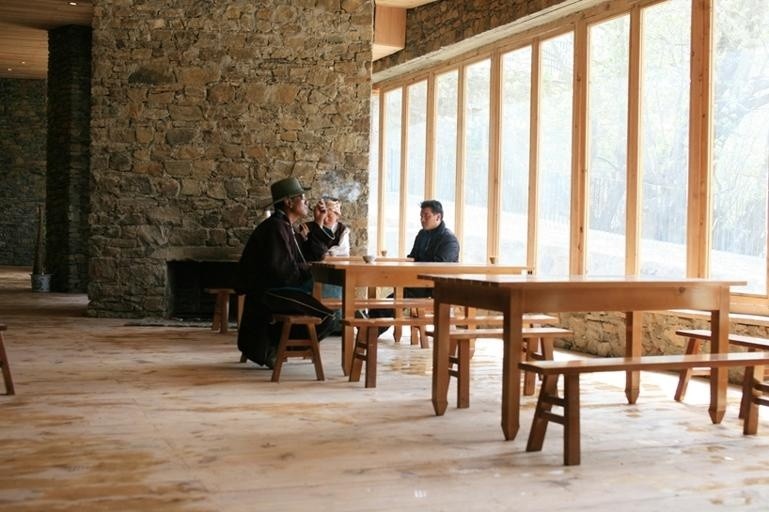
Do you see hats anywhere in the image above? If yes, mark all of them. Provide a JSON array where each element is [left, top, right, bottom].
[[261, 177, 312, 208]]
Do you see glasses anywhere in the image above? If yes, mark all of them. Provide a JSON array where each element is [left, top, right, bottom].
[[291, 193, 304, 199]]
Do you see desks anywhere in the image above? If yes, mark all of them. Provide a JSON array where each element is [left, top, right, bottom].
[[417, 274, 747, 441], [313, 261, 528, 375], [311, 257, 415, 342]]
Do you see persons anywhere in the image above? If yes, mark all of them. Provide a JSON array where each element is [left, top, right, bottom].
[[302, 199, 352, 257], [355, 201, 461, 339], [235, 173, 337, 368]]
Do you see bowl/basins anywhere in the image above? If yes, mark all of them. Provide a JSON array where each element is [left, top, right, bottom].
[[362, 255, 375, 264]]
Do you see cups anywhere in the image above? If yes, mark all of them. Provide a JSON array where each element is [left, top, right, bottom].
[[328, 250, 337, 257]]
